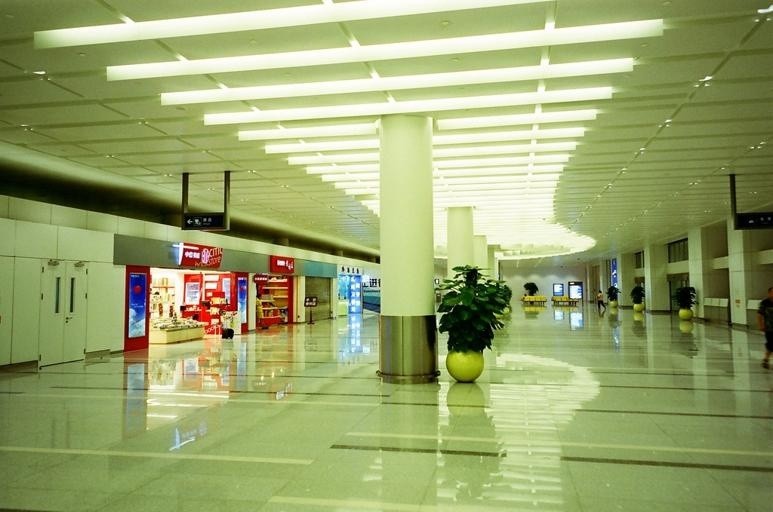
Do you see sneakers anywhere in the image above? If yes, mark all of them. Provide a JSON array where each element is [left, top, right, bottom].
[[760, 359, 769, 369]]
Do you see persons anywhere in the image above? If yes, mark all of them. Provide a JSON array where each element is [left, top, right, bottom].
[[755, 287, 772, 370], [596, 289, 606, 310], [255, 292, 264, 330], [596, 310, 605, 329]]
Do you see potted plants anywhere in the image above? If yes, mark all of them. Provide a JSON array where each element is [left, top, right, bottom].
[[606, 285, 644, 312], [671, 287, 698, 320], [433, 262, 513, 386]]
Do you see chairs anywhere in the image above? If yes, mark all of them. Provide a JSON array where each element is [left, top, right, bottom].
[[521, 295, 580, 306]]
